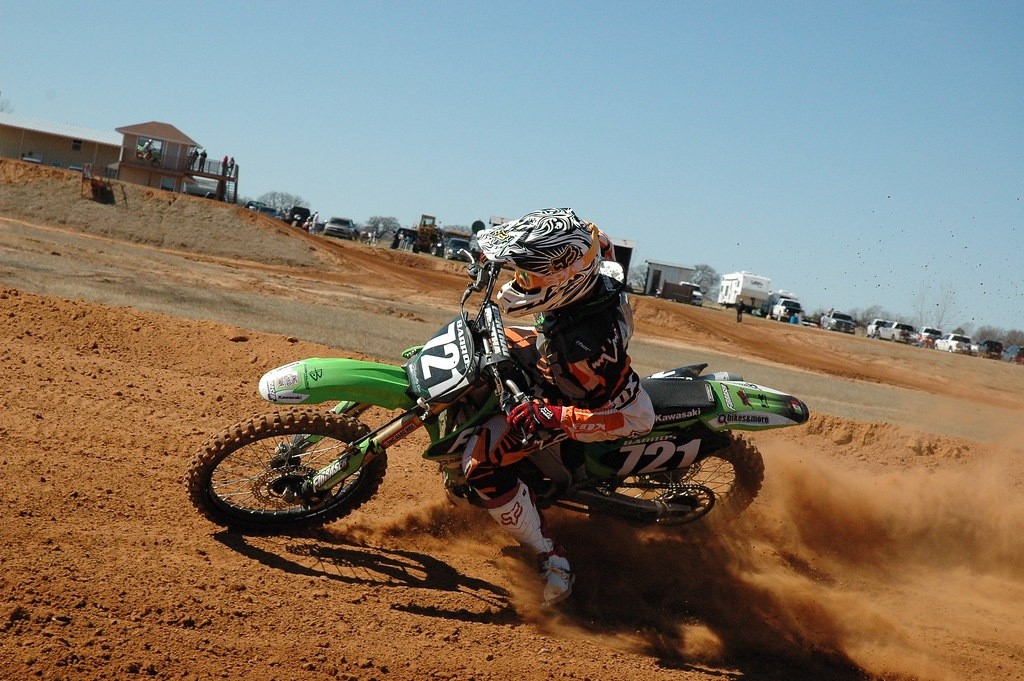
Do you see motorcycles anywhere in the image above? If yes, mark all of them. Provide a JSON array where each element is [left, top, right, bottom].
[[181, 244, 811, 532]]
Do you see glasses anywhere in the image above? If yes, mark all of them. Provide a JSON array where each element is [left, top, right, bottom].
[[511, 263, 534, 289]]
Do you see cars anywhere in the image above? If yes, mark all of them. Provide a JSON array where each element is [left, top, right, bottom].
[[865, 319, 917, 345], [970, 339, 1003, 359], [820, 308, 856, 335], [678, 281, 706, 305], [430, 237, 474, 261], [324, 217, 355, 241], [933, 333, 972, 354], [288, 206, 311, 228], [1001, 345, 1024, 365], [919, 326, 942, 340]]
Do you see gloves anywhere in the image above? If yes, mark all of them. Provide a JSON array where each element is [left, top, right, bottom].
[[480, 253, 488, 263], [506, 402, 562, 442]]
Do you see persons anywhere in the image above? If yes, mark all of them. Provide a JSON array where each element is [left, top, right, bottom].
[[144, 139, 155, 161], [189, 146, 200, 172], [222, 157, 235, 176], [303, 210, 320, 235], [474, 204, 655, 604], [736, 297, 744, 324], [399, 231, 410, 250], [198, 150, 207, 171]]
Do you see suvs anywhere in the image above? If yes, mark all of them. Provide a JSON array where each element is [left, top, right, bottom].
[[389, 229, 425, 252]]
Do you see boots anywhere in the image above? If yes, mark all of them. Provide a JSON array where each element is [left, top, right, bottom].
[[483, 476, 576, 605]]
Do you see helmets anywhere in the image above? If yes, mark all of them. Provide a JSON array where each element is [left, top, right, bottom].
[[476, 207, 602, 317]]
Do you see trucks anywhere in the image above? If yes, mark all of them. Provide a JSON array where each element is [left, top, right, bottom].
[[761, 291, 804, 323]]
